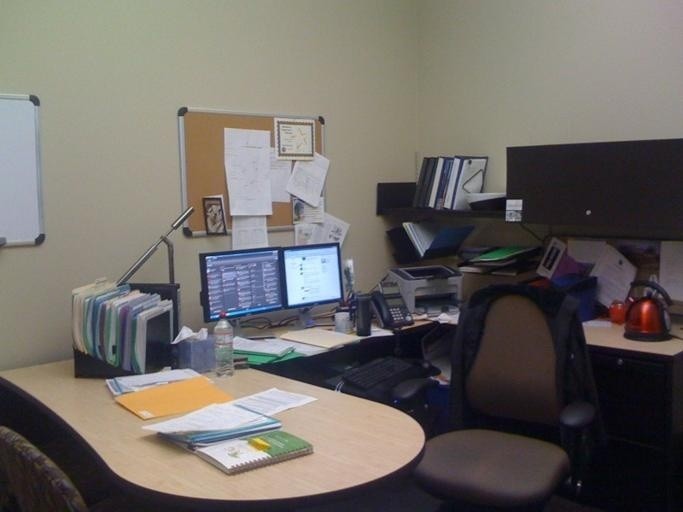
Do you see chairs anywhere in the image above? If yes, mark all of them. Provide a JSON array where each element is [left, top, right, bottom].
[[387, 282, 602, 511]]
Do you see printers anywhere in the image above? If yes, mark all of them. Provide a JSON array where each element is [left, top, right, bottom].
[[387, 264, 464, 313]]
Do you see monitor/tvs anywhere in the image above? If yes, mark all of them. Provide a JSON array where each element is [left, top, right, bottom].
[[199, 247, 283, 339], [280, 242, 344, 327], [505, 138, 683, 241]]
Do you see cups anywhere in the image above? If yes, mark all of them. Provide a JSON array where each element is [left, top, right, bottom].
[[609, 302, 626, 324], [333, 312, 353, 333]]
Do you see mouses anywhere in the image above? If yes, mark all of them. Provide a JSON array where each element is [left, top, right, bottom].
[[420, 359, 432, 373]]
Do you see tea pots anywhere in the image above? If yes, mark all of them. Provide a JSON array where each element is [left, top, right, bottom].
[[622, 278, 676, 341]]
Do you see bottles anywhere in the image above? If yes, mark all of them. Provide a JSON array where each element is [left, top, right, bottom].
[[213, 311, 235, 377], [356, 295, 372, 336]]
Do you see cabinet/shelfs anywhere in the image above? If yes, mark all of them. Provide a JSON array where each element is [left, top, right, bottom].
[[585, 345, 682, 511]]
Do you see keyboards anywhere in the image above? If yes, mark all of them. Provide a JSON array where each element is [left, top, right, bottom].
[[341, 355, 412, 390]]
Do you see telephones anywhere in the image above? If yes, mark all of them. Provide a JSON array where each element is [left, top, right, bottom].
[[371, 282, 414, 328]]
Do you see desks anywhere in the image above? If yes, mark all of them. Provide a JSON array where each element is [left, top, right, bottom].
[[0, 309, 431, 510]]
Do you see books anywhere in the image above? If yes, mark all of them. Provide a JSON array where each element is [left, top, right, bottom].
[[104, 365, 282, 453], [68, 276, 174, 375], [196, 429, 314, 477], [232, 326, 358, 364], [402, 219, 539, 276]]
